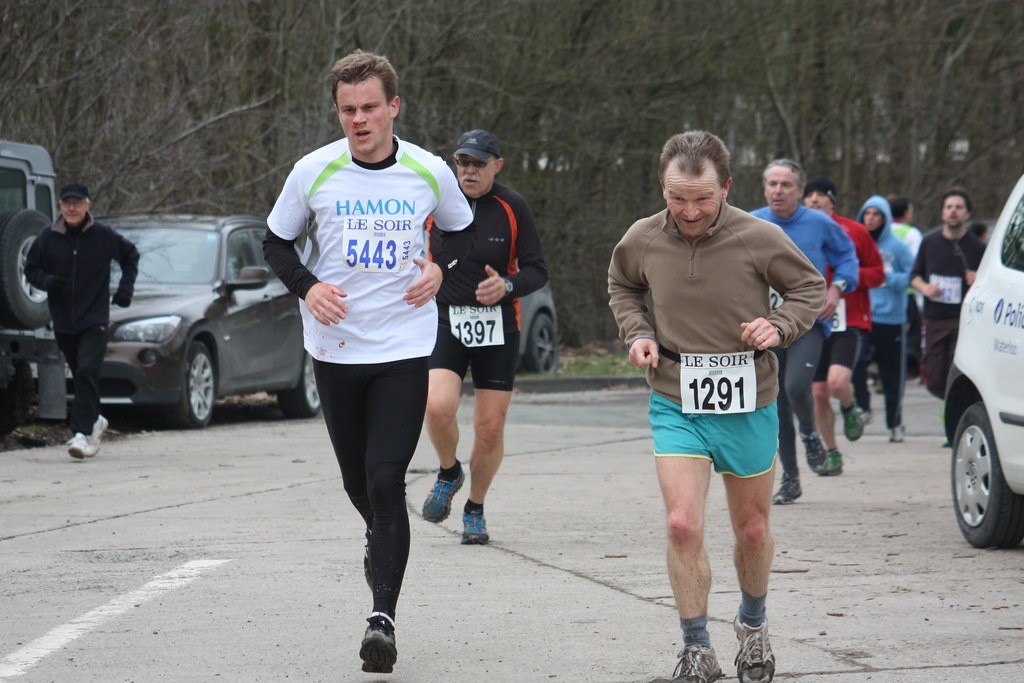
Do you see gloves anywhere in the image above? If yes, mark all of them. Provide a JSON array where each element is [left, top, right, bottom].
[[112, 293, 131, 308], [44, 275, 67, 295]]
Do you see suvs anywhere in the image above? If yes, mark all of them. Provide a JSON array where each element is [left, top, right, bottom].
[[0, 139, 62, 442], [28, 214, 322, 430]]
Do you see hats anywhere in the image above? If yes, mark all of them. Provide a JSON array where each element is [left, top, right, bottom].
[[804, 178, 837, 207], [455, 129, 501, 164], [60, 183, 90, 202]]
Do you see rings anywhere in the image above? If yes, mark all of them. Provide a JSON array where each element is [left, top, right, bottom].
[[760, 335, 763, 339]]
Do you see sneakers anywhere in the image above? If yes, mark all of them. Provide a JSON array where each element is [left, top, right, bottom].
[[363, 527, 374, 591], [670, 644, 722, 683], [360, 623, 396, 673], [460, 501, 490, 546], [423, 467, 465, 523], [799, 428, 825, 473], [86, 415, 109, 457], [66, 432, 85, 459], [823, 448, 842, 475], [731, 610, 775, 683], [839, 399, 863, 440], [770, 470, 800, 507]]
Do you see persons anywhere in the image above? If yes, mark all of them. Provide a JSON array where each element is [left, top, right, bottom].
[[803, 176, 885, 476], [852, 196, 914, 443], [909, 189, 988, 448], [22, 183, 141, 459], [262, 53, 473, 673], [424, 129, 548, 545], [970, 222, 988, 240], [607, 131, 826, 683], [748, 159, 859, 505], [889, 194, 923, 380]]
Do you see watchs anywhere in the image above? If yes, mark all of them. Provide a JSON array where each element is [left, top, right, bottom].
[[832, 280, 846, 291], [505, 279, 513, 296], [775, 325, 783, 335]]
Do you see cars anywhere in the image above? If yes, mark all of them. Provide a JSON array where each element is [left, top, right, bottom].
[[941, 175, 1024, 553], [513, 280, 560, 377]]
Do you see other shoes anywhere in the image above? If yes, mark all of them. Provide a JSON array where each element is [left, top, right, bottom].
[[857, 406, 874, 426], [891, 423, 906, 443]]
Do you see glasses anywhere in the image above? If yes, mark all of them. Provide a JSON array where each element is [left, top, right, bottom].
[[455, 159, 495, 169]]
